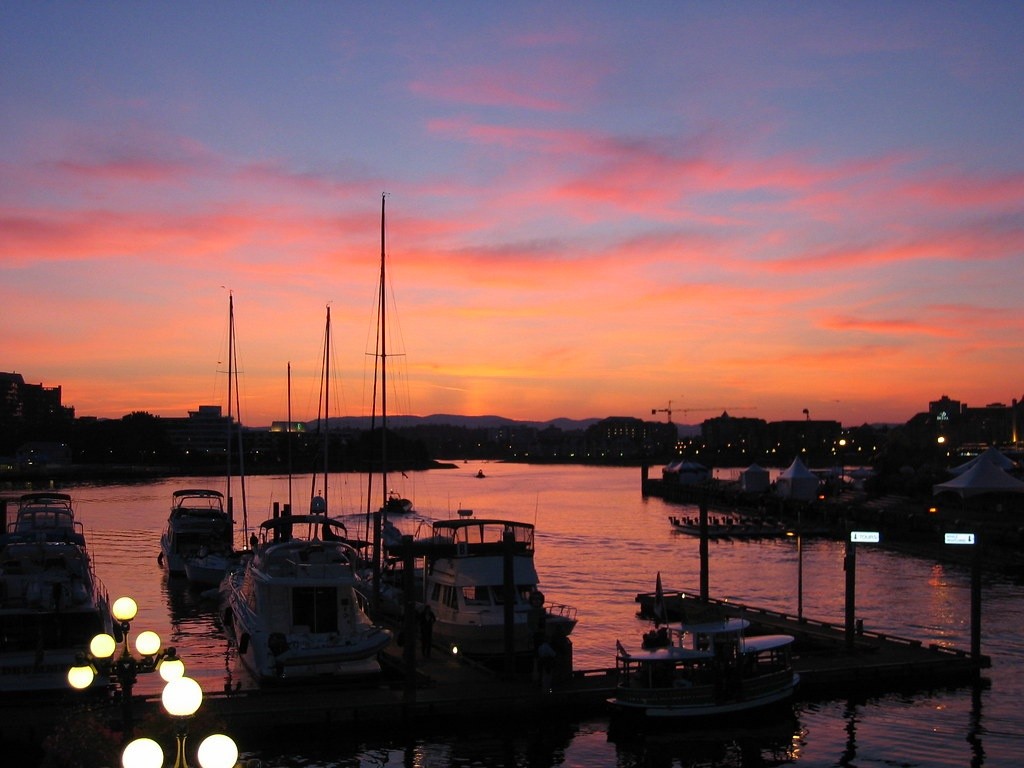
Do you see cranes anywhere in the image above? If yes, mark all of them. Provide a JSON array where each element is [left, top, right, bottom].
[[651, 399, 760, 424]]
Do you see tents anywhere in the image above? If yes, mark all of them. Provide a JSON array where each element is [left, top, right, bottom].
[[666, 442, 1024, 515]]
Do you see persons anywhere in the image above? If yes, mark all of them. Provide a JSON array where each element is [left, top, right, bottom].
[[400, 600, 437, 657], [250, 533, 258, 550]]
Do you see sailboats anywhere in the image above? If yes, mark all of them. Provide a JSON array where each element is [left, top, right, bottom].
[[150, 191, 581, 694]]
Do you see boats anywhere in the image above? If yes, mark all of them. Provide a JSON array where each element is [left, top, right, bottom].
[[604, 618, 808, 753], [0, 490, 125, 662]]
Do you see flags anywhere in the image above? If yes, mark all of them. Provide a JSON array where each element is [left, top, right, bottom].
[[652, 574, 664, 627]]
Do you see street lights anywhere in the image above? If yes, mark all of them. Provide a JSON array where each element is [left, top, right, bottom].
[[69, 597, 178, 742]]
